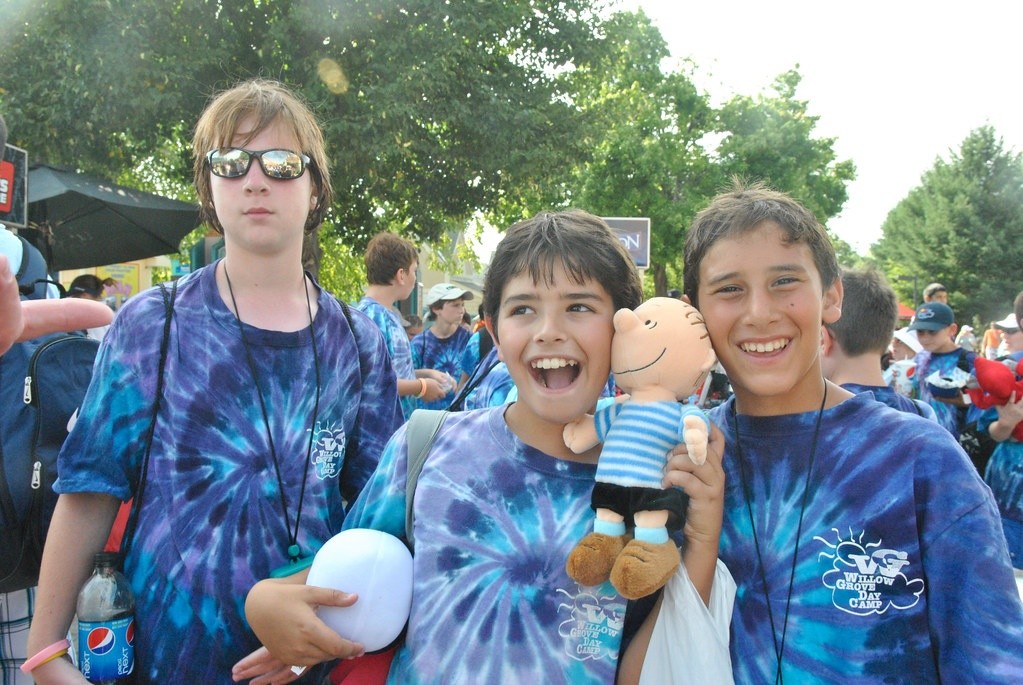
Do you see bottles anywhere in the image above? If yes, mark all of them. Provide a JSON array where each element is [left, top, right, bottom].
[[78, 552, 137, 685]]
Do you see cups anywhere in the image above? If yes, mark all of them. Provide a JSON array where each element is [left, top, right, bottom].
[[892, 360, 918, 398]]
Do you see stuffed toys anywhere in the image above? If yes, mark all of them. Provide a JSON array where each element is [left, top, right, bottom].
[[560, 296, 718, 600], [968, 356, 1023, 442]]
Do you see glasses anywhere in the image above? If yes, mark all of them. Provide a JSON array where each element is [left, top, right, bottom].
[[1007, 328, 1021, 334], [204, 146, 316, 180]]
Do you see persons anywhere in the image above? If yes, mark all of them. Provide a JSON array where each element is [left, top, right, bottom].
[[26, 82, 404, 685], [684, 187, 1023, 685], [0, 114, 63, 685], [340, 232, 517, 417], [820, 264, 1023, 569], [245, 206, 727, 685], [1, 255, 114, 359]]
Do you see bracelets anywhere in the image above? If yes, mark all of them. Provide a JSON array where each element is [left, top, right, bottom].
[[19, 639, 70, 673], [414, 378, 426, 399]]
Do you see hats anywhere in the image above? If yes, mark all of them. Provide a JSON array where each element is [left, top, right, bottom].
[[427, 282, 474, 305], [64, 282, 100, 296], [906, 302, 953, 333], [894, 327, 924, 353], [993, 313, 1021, 329]]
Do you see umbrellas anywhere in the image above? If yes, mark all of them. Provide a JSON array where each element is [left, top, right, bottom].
[[9, 161, 204, 272]]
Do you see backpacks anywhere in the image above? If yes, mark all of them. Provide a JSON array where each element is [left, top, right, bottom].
[[0, 230, 103, 589]]
